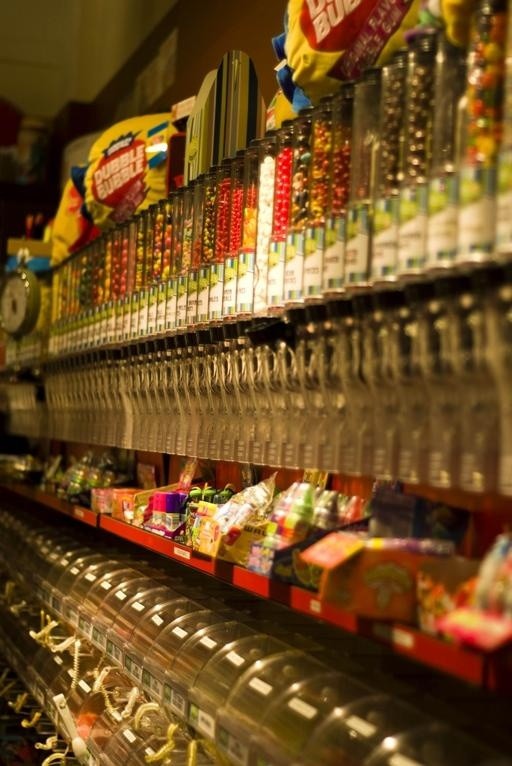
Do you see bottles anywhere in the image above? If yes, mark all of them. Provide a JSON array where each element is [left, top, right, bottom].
[[468, 531, 511, 614], [57, 449, 118, 505], [264, 482, 370, 542], [0, 0, 512, 365], [188, 488, 230, 512]]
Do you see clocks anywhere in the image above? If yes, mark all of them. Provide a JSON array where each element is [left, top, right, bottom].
[[0, 267, 42, 340]]
